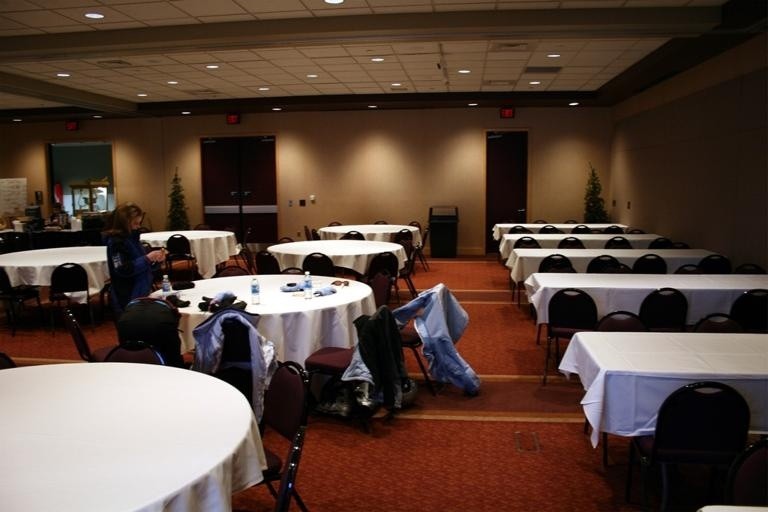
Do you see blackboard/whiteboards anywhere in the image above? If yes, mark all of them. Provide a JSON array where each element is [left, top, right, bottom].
[[0, 177, 27, 219]]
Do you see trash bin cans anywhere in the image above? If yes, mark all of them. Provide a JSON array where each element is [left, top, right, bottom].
[[428, 206, 459, 258]]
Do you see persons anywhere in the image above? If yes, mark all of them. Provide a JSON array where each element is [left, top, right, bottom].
[[101, 201, 166, 321], [50, 204, 68, 224], [70, 211, 82, 230]]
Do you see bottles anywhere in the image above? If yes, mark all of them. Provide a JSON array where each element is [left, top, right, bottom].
[[303, 271, 312, 300], [162, 275, 169, 301], [251, 276, 259, 305]]
